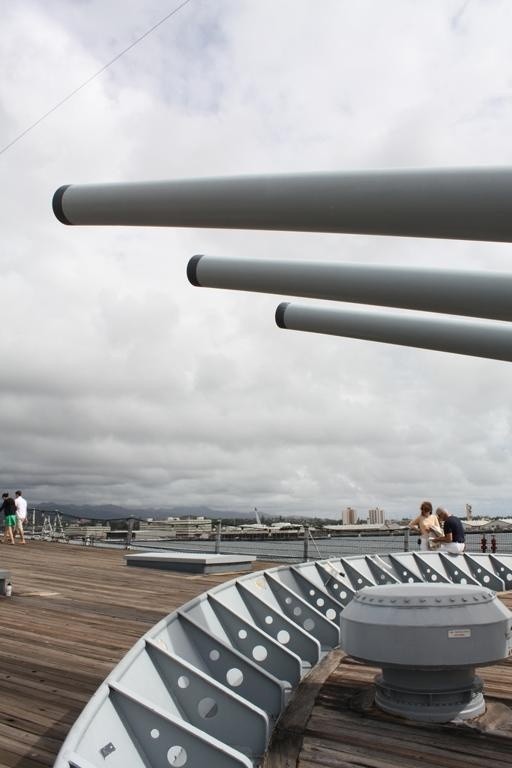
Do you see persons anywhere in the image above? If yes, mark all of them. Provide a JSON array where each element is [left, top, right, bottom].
[[407, 500, 467, 554], [0, 490, 29, 545]]
